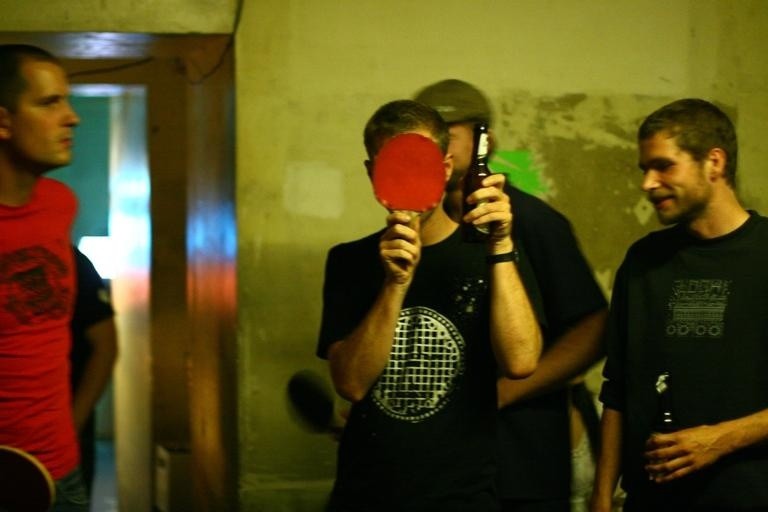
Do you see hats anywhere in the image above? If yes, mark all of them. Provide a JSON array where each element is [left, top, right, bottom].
[[416, 79, 492, 127]]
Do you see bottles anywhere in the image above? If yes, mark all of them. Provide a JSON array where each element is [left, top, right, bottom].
[[650, 366, 685, 486], [464, 121, 498, 245]]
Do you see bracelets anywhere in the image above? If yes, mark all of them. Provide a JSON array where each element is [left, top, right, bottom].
[[487, 247, 521, 266]]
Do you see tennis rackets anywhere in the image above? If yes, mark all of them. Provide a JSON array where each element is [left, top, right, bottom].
[[0, 446, 57, 510], [372, 133, 447, 241], [283, 369, 347, 440]]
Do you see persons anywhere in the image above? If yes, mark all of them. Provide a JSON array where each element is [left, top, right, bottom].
[[587, 96, 766, 511], [410, 77, 611, 511], [64, 239, 118, 512], [1, 44, 93, 511], [313, 99, 544, 508]]
